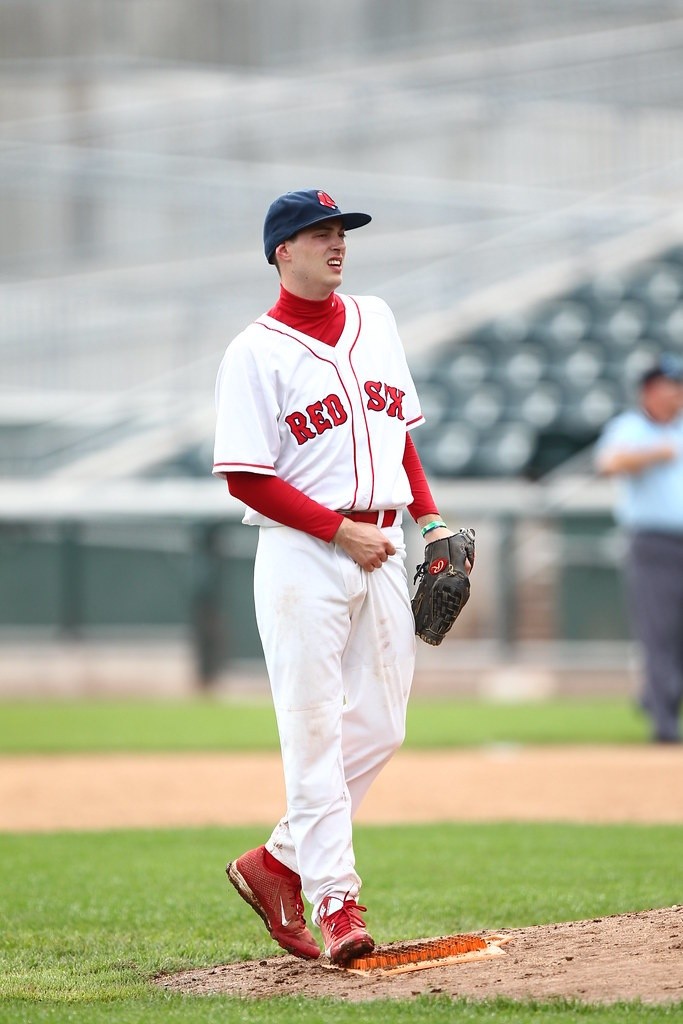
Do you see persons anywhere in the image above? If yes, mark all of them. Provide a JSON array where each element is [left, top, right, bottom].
[[212, 190, 475, 962], [592, 355, 683, 745]]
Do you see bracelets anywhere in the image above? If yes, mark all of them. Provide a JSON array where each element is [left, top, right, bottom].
[[421, 521, 447, 538]]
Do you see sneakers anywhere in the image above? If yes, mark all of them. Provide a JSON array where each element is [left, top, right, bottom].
[[226, 844, 321, 960], [319, 890, 375, 966]]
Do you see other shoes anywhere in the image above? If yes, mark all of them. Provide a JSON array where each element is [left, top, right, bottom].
[[655, 719, 683, 744]]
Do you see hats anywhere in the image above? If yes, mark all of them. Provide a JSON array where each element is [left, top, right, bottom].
[[622, 348, 683, 398], [263, 190, 372, 265]]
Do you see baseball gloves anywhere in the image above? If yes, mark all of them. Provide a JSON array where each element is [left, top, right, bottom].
[[411, 529, 477, 647]]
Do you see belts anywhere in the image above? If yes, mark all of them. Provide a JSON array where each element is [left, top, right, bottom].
[[340, 509, 396, 528]]
